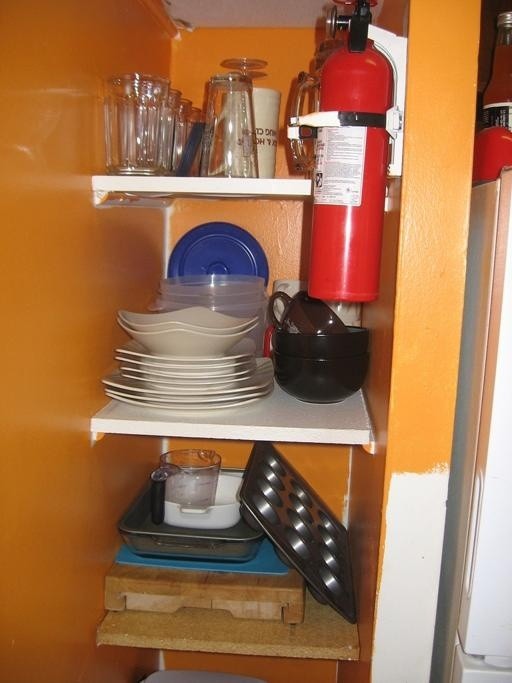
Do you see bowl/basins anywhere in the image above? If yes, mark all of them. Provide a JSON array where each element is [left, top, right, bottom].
[[272, 281, 361, 326], [158, 475, 247, 526], [271, 327, 370, 403]]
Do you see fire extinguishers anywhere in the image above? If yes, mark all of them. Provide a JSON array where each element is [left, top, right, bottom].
[[308, 0, 396, 302]]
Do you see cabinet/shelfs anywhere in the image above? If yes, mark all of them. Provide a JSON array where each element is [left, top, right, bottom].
[[1, 0, 482, 683]]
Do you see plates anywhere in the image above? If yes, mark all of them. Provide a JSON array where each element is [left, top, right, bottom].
[[120, 478, 260, 561], [99, 307, 273, 408], [238, 442, 357, 626]]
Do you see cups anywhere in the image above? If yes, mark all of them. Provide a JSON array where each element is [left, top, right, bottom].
[[152, 449, 221, 510], [210, 85, 280, 177], [103, 74, 161, 177], [267, 291, 347, 334], [165, 88, 199, 176], [202, 74, 259, 177], [284, 61, 323, 174]]
